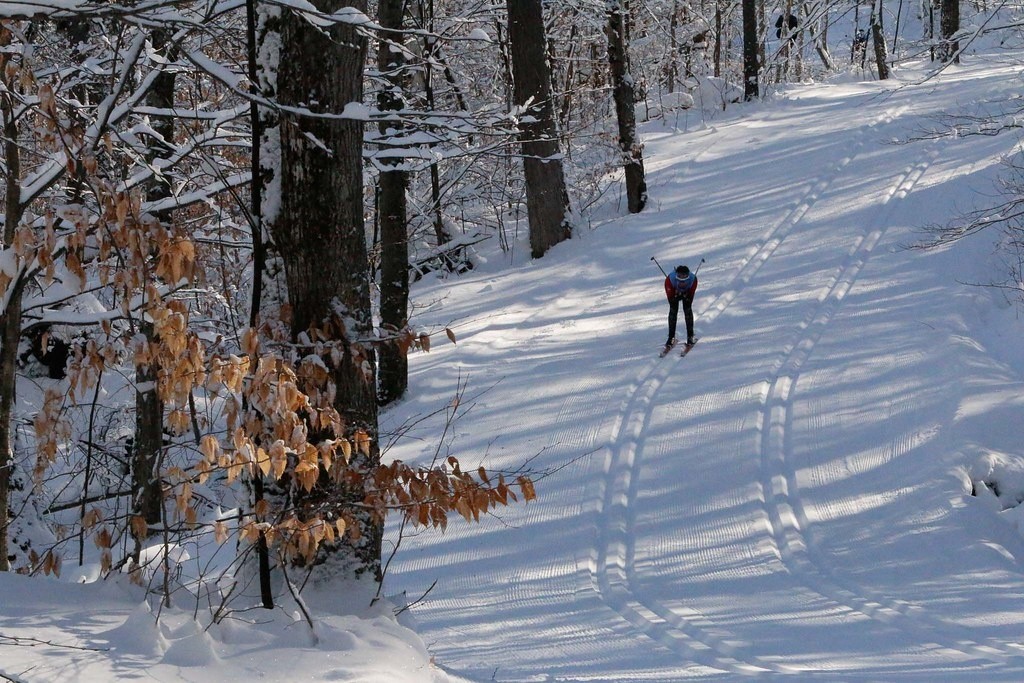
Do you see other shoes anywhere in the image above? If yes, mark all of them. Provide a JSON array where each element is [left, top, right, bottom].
[[666, 337, 675, 348], [687, 337, 694, 346]]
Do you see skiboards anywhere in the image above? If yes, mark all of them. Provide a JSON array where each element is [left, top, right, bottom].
[[658, 338, 699, 358]]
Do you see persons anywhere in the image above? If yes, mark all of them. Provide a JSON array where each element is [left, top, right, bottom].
[[664, 266, 698, 347]]
